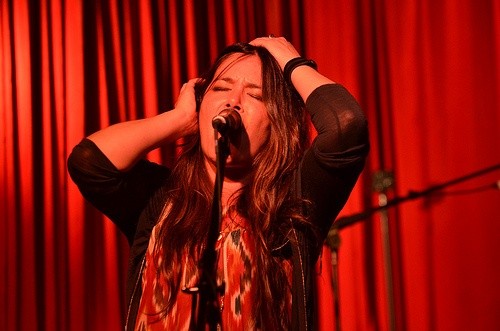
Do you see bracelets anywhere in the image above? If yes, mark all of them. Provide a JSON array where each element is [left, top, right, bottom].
[[284, 53, 317, 83]]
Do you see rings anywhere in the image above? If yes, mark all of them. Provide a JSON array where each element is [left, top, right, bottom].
[[268, 34, 276, 39]]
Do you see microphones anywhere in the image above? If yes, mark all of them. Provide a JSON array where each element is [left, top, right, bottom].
[[211, 108, 242, 133]]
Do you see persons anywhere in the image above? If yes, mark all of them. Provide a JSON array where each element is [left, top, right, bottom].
[[68, 36, 370, 330]]
